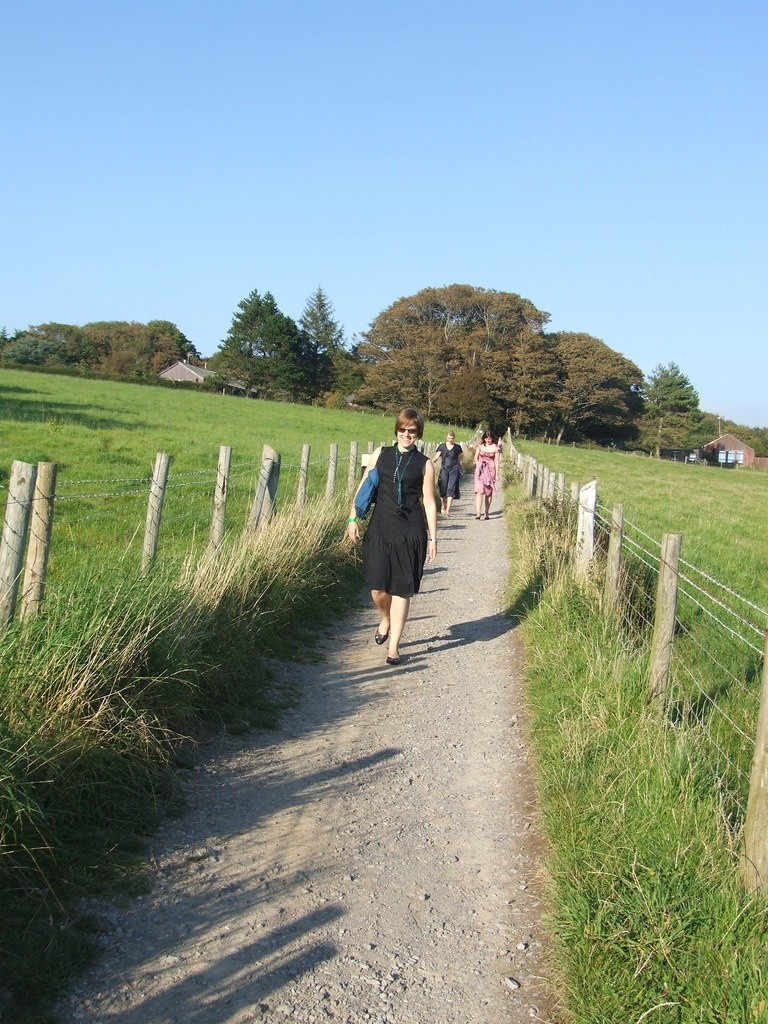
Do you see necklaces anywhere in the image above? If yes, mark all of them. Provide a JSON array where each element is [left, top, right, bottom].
[[392, 442, 415, 506]]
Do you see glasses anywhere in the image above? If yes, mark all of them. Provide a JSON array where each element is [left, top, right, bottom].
[[398, 428, 417, 434]]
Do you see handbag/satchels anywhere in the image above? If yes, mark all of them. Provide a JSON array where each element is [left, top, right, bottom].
[[353, 447, 384, 519]]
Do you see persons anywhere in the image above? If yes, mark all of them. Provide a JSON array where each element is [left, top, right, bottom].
[[432, 429, 464, 516], [474, 431, 500, 520], [344, 407, 438, 664]]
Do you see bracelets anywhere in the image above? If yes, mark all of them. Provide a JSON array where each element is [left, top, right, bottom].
[[346, 518, 358, 523], [430, 538, 436, 543]]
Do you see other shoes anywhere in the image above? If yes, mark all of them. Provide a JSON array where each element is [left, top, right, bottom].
[[446, 508, 450, 516], [441, 505, 446, 515], [375, 626, 388, 645], [475, 513, 481, 519], [485, 513, 489, 520], [387, 654, 400, 665]]
